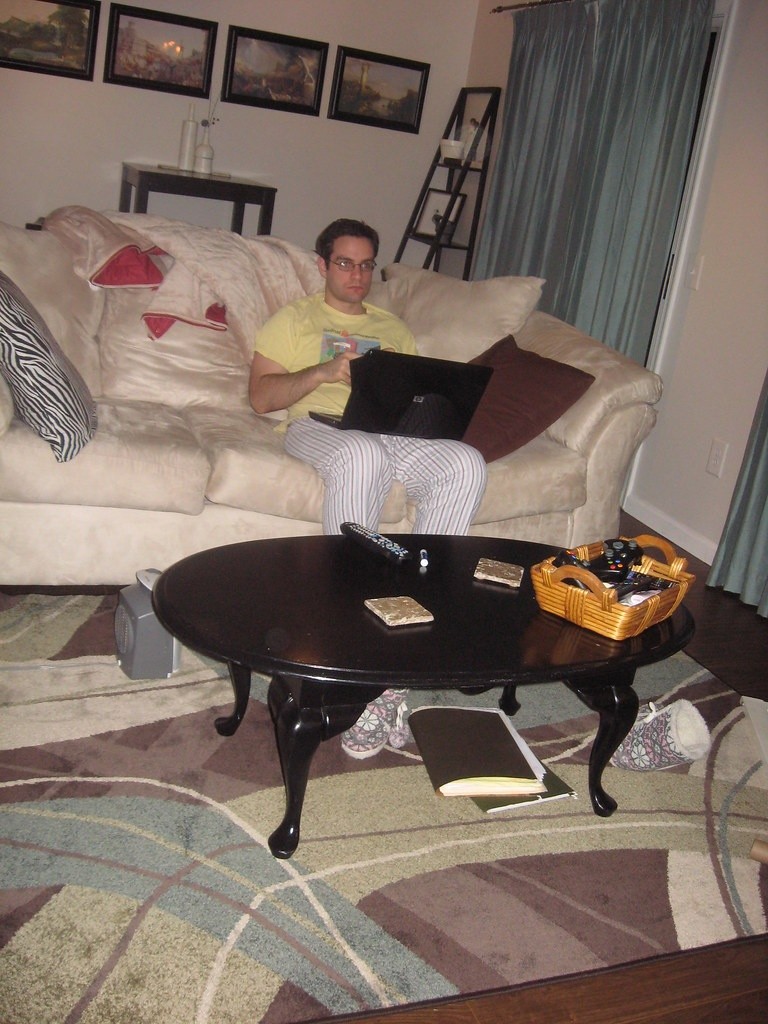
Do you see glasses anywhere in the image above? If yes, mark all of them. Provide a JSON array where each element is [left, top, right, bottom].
[[322, 256, 376, 272]]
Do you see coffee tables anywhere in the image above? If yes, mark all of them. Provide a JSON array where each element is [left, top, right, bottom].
[[151, 533, 696, 858]]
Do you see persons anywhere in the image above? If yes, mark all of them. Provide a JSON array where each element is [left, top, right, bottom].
[[248, 218, 488, 535]]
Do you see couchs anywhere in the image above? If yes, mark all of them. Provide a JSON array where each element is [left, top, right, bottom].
[[0, 208, 662, 584]]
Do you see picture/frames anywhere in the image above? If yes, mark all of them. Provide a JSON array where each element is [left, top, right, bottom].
[[327, 45, 432, 135], [104, 3, 217, 99], [221, 25, 329, 117], [413, 189, 467, 244], [1, 0, 101, 82]]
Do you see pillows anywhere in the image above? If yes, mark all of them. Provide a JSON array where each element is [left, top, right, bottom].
[[383, 262, 597, 467], [0, 273, 96, 463]]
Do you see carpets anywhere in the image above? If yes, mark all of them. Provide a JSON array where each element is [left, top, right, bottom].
[[1, 595, 768, 1024]]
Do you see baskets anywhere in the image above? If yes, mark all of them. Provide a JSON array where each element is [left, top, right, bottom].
[[531, 535, 696, 641]]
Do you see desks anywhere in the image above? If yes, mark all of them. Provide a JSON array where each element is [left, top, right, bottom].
[[117, 163, 278, 235]]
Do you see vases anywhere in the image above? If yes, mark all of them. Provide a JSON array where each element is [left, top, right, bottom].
[[440, 139, 464, 165], [194, 126, 215, 173], [436, 223, 454, 245]]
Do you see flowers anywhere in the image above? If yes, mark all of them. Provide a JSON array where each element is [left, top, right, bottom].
[[201, 116, 220, 126]]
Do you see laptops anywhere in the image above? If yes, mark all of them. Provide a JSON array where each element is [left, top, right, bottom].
[[308, 350, 495, 440]]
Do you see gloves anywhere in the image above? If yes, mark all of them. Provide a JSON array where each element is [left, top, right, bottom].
[[609, 698, 712, 771], [340, 686, 409, 760]]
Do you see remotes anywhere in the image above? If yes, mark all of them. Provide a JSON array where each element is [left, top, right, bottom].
[[340, 522, 411, 567]]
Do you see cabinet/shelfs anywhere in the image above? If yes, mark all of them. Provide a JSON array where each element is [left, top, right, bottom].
[[395, 85, 502, 282]]
